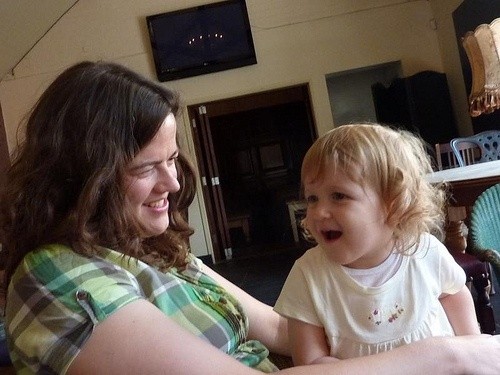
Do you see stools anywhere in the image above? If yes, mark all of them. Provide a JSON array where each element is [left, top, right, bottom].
[[450, 251, 496, 295], [286, 199, 308, 243]]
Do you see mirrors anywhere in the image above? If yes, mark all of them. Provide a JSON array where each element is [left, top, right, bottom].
[[325, 60, 404, 127]]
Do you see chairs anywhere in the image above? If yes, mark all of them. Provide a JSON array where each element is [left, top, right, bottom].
[[470, 183, 500, 285], [451, 130, 500, 167]]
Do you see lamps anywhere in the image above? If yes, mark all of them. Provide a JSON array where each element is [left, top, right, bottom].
[[461, 17, 500, 118]]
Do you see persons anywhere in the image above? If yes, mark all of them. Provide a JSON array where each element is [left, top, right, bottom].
[[0, 61, 500, 375], [273, 123, 480, 366]]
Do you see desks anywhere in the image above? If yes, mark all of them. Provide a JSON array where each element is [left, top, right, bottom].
[[427, 159, 500, 187], [227, 214, 252, 242]]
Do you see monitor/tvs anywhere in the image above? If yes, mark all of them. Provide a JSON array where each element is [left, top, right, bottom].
[[146, 0, 257, 82]]
